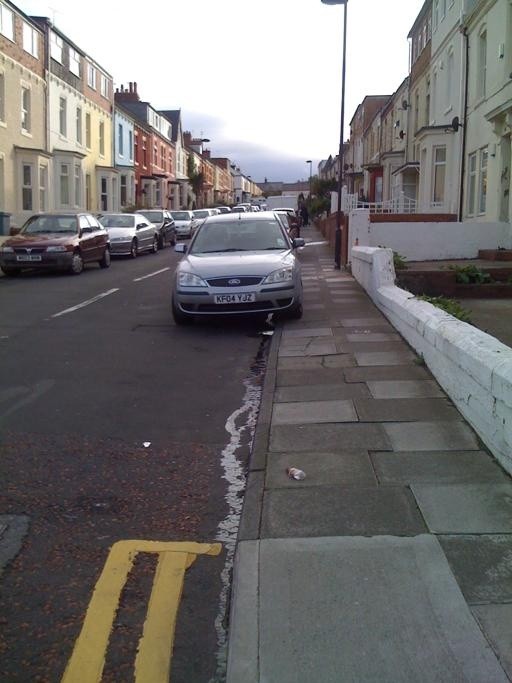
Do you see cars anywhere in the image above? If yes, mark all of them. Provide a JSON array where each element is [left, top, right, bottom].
[[95, 195, 298, 259], [0, 212, 111, 277], [172, 212, 303, 323]]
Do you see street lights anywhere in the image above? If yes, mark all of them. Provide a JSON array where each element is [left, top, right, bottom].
[[304, 159, 313, 206], [321, 0, 346, 269]]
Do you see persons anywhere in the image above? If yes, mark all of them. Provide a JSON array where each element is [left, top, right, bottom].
[[300, 206, 308, 227]]
